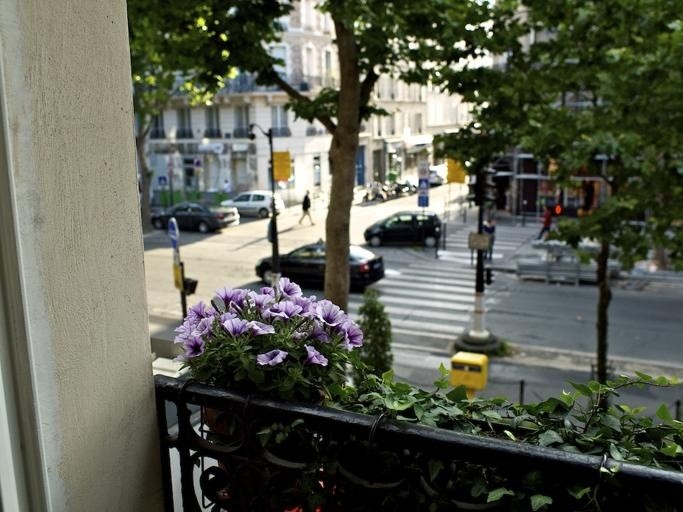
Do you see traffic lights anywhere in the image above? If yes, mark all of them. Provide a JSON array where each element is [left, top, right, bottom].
[[467, 162, 497, 206], [553, 205, 563, 214]]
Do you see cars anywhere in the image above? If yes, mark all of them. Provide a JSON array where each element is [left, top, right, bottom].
[[615, 220, 673, 241], [429, 170, 442, 185], [365, 211, 441, 246], [221, 191, 285, 219], [255, 240, 384, 291], [152, 199, 240, 235]]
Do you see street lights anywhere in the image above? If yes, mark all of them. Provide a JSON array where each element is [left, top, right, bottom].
[[248, 123, 278, 279]]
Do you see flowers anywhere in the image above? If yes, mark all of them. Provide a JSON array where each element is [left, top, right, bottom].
[[173, 276, 364, 391]]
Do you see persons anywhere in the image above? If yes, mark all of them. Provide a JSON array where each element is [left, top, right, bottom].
[[298, 190, 315, 225], [535, 204, 553, 240], [483, 218, 495, 261]]
